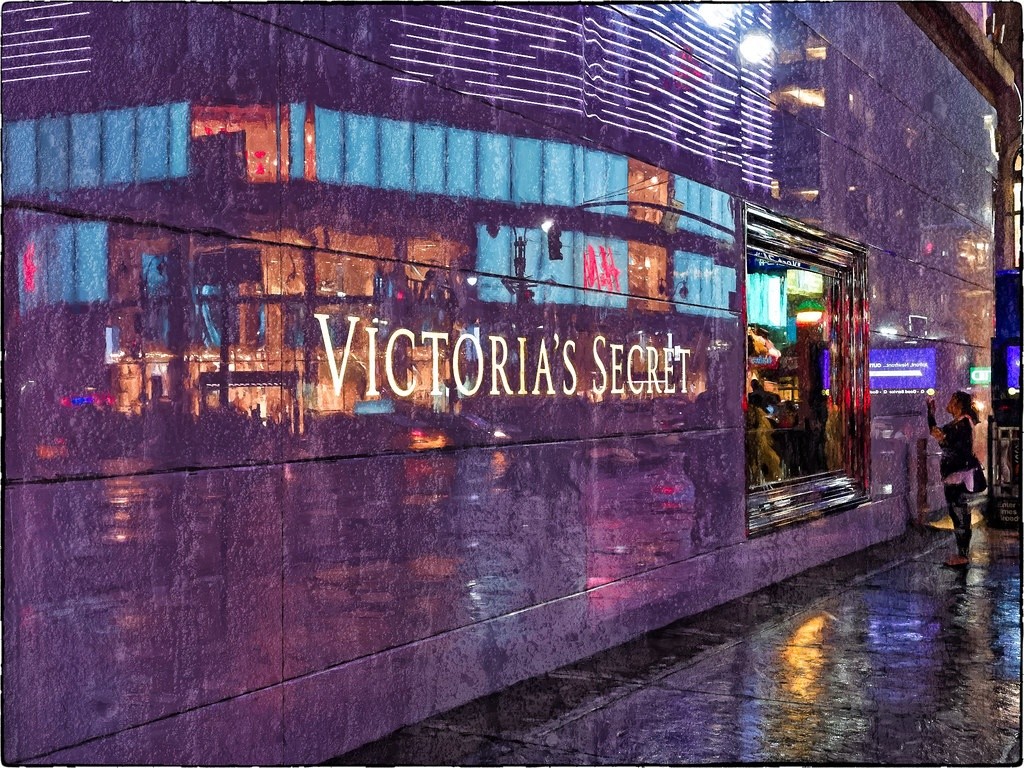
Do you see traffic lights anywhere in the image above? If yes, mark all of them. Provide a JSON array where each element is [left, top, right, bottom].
[[549, 219, 564, 262]]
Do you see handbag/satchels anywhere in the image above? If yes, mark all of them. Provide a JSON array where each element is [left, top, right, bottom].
[[964, 453, 986, 494]]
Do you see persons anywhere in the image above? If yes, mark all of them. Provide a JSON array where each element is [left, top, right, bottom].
[[926, 390, 982, 564]]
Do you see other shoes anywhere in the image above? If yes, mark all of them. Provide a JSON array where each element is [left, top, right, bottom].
[[950, 552, 970, 558], [942, 558, 969, 566]]
